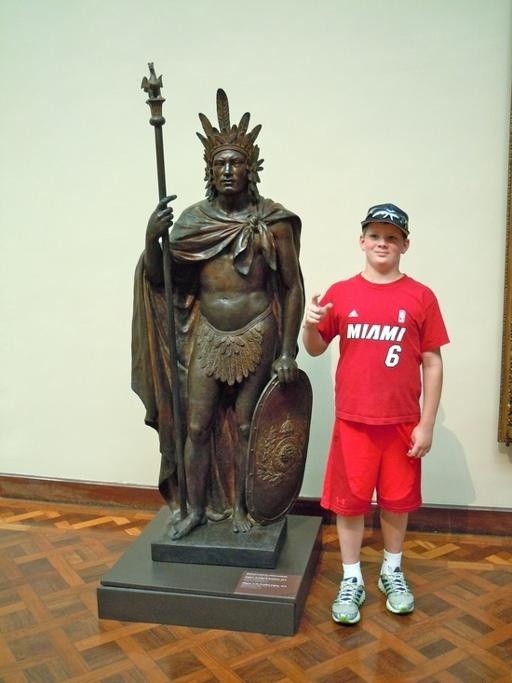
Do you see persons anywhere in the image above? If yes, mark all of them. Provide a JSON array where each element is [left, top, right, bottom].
[[301, 204, 451, 623], [131, 89, 305, 541]]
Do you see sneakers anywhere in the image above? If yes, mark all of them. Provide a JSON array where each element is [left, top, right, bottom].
[[331, 576, 366, 624], [377, 566, 414, 614]]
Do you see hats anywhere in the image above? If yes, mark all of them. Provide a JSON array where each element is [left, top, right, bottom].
[[360, 202, 409, 236]]
